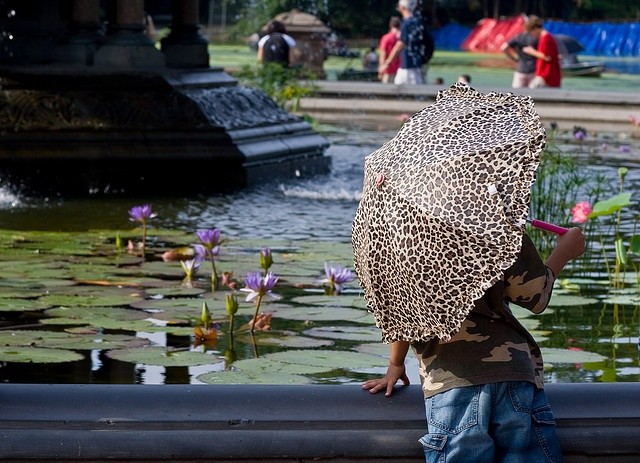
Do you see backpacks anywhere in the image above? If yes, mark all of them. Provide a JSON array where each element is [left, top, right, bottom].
[[263, 32, 290, 66]]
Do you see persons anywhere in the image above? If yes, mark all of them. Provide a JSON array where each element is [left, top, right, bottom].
[[362, 227, 587, 461], [523, 15, 562, 88], [256, 20, 302, 66], [455, 74, 470, 85], [379, 0, 427, 84], [377, 17, 401, 80], [499, 29, 538, 88]]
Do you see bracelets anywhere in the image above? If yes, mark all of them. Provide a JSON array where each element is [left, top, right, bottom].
[[384, 59, 392, 64]]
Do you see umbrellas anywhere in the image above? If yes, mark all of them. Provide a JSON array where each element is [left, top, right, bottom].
[[350, 82, 568, 347]]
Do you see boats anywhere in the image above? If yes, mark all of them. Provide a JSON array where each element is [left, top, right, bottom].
[[560, 60, 607, 78], [328, 49, 361, 58], [335, 69, 380, 83]]
